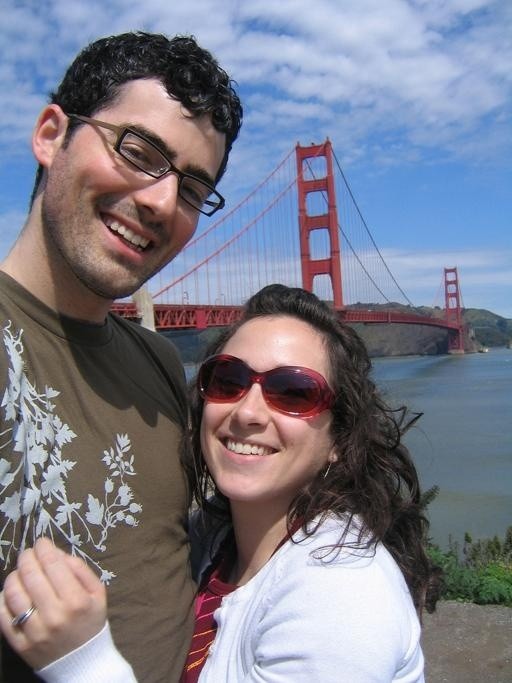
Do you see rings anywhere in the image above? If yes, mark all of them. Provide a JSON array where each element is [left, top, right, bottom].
[[12, 604, 39, 624]]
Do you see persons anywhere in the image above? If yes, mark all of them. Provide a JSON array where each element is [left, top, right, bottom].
[[2, 26, 247, 683], [0, 279, 427, 682]]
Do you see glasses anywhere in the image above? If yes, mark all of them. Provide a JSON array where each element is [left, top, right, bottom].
[[196, 351, 339, 421], [63, 106, 224, 218]]
